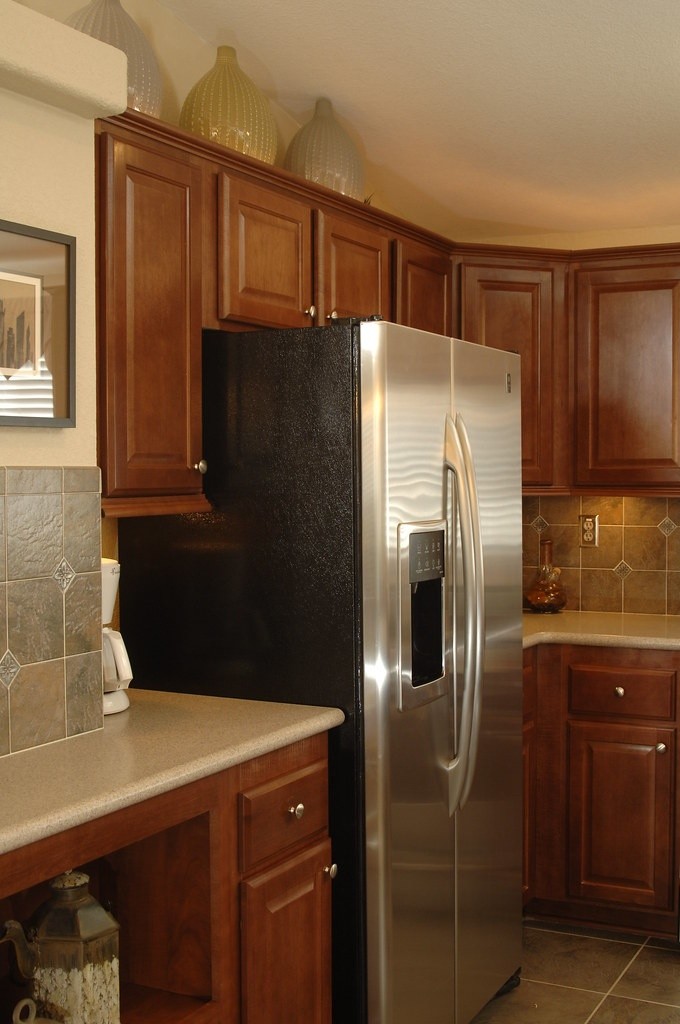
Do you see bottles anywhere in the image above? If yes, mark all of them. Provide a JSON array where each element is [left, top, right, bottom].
[[527, 539, 567, 613]]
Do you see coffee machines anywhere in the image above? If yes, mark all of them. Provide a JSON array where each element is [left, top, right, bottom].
[[96, 557, 134, 715]]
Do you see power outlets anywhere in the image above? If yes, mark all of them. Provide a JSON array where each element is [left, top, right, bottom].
[[579, 515, 600, 547]]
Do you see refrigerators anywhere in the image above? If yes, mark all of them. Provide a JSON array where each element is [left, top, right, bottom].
[[117, 316, 527, 1024]]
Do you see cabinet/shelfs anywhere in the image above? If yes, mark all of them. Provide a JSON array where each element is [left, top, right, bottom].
[[456, 242, 570, 497], [522, 643, 680, 942], [94, 107, 210, 518], [0, 732, 344, 1024], [571, 242, 680, 498], [211, 141, 391, 333], [392, 215, 453, 337]]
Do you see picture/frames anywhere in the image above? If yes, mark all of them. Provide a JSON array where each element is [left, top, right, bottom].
[[0, 220, 76, 428]]
